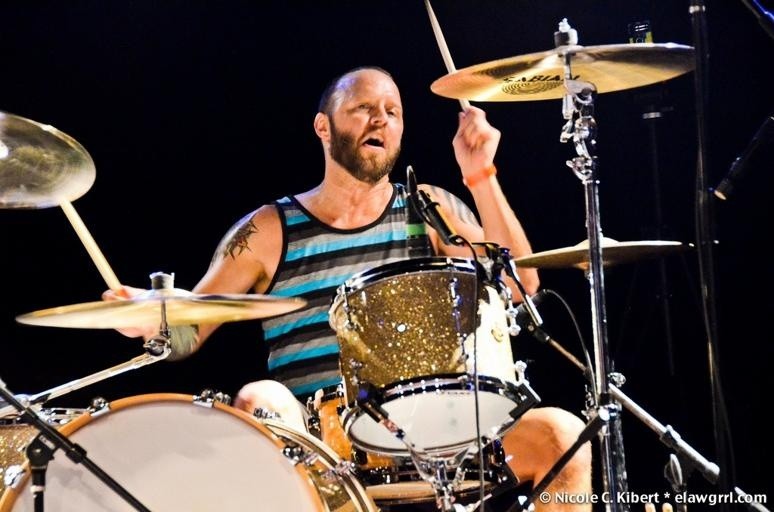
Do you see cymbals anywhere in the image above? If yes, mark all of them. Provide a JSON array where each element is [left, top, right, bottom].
[[513, 238, 719, 270], [15, 288, 307, 329], [429, 43, 710, 103], [0, 113, 98, 210]]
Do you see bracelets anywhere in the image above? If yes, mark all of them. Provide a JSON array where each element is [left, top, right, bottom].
[[462, 164, 498, 186]]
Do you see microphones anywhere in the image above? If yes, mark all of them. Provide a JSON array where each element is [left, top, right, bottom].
[[505, 288, 547, 326], [405, 166, 432, 260]]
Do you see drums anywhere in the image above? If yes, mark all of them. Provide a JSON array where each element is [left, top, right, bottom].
[[306, 384, 495, 476], [0, 403, 96, 497], [329, 257, 524, 455], [0, 389, 382, 512], [366, 482, 501, 512]]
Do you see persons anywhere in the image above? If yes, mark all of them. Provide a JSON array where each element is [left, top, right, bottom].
[[101, 66, 593, 512]]
